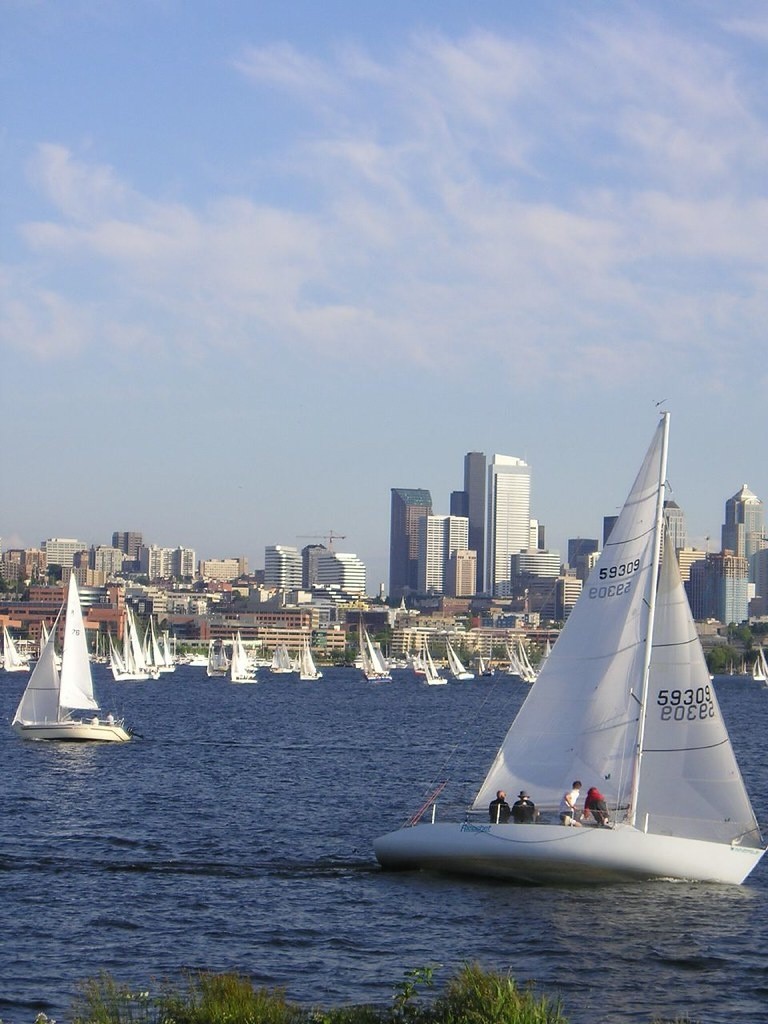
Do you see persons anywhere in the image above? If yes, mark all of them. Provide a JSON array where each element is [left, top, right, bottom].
[[559, 780, 582, 828], [584, 787, 609, 826], [489, 790, 510, 824], [92, 714, 99, 725], [510, 791, 541, 824], [106, 711, 114, 725]]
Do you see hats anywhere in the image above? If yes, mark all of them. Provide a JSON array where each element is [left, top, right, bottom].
[[518, 791, 531, 798]]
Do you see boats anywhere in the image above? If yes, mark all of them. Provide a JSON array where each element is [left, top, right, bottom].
[[175, 652, 209, 667]]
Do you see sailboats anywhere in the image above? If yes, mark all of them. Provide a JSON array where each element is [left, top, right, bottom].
[[205, 641, 231, 677], [295, 634, 323, 680], [88, 602, 179, 683], [505, 637, 555, 684], [359, 618, 391, 683], [422, 636, 448, 685], [11, 568, 134, 742], [230, 629, 258, 684], [0, 625, 32, 672], [412, 651, 425, 675], [477, 652, 494, 676], [373, 400, 767, 886], [268, 632, 298, 674], [36, 620, 62, 672], [751, 642, 767, 682], [446, 639, 476, 681]]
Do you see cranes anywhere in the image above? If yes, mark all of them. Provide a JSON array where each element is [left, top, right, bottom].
[[297, 530, 346, 551]]
